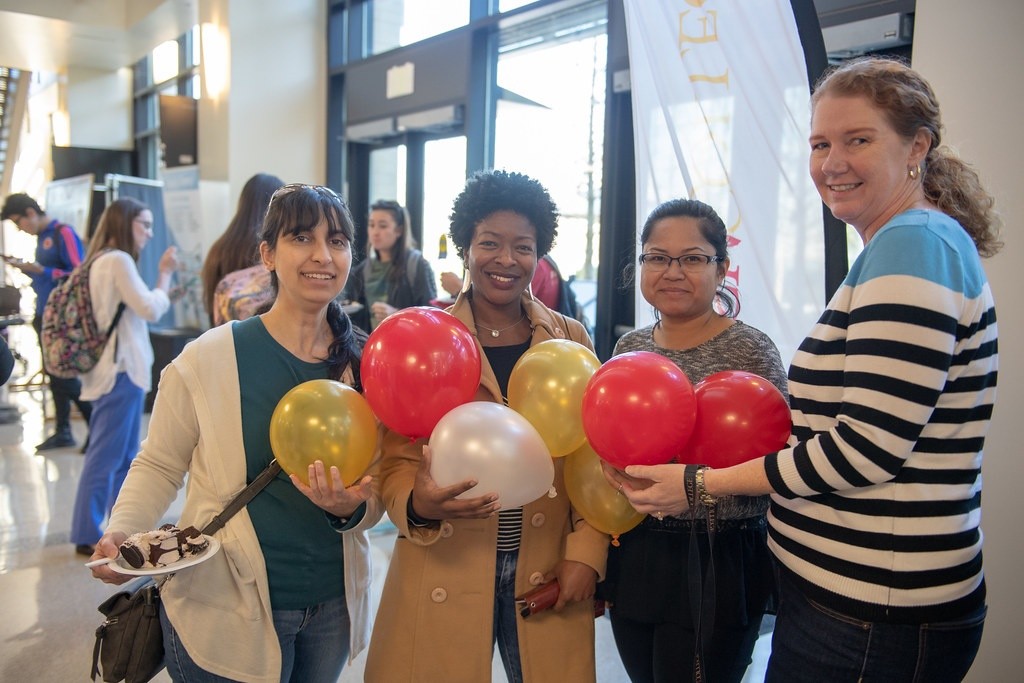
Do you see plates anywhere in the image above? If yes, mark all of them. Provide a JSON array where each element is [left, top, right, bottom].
[[107, 537, 220, 575]]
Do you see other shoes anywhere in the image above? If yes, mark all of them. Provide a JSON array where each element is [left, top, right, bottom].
[[36, 433, 74, 450], [76, 543, 95, 555]]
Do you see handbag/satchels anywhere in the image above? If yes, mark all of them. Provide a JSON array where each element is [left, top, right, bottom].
[[91, 576, 167, 683]]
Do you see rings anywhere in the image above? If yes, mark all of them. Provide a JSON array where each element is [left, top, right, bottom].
[[619, 484, 622, 492], [658, 511, 663, 521]]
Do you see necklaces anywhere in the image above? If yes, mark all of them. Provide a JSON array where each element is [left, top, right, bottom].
[[474, 315, 525, 337]]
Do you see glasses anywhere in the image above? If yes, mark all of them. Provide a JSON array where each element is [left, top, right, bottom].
[[639, 253, 722, 273], [133, 216, 152, 228], [15, 214, 23, 224], [268, 184, 348, 217]]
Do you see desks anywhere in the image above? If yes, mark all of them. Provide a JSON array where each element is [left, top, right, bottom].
[[0, 315, 52, 387]]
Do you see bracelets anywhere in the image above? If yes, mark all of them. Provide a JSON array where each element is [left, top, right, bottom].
[[684, 464, 719, 683]]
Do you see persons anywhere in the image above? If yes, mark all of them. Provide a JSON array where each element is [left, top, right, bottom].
[[0, 193, 93, 453], [0, 333, 15, 386], [67, 197, 179, 557], [622, 56, 1004, 683], [440, 254, 561, 311], [89, 184, 386, 683], [200, 174, 286, 329], [606, 199, 790, 683], [351, 200, 437, 336], [363, 171, 611, 683]]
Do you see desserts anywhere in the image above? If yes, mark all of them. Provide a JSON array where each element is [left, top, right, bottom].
[[119, 524, 211, 568]]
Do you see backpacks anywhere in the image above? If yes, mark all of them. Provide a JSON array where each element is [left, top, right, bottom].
[[542, 254, 591, 335], [40, 247, 126, 378]]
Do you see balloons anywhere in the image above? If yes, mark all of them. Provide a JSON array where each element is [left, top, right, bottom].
[[427, 401, 555, 511], [506, 339, 603, 457], [360, 306, 482, 440], [583, 350, 792, 470], [564, 441, 649, 546], [269, 379, 376, 489]]
